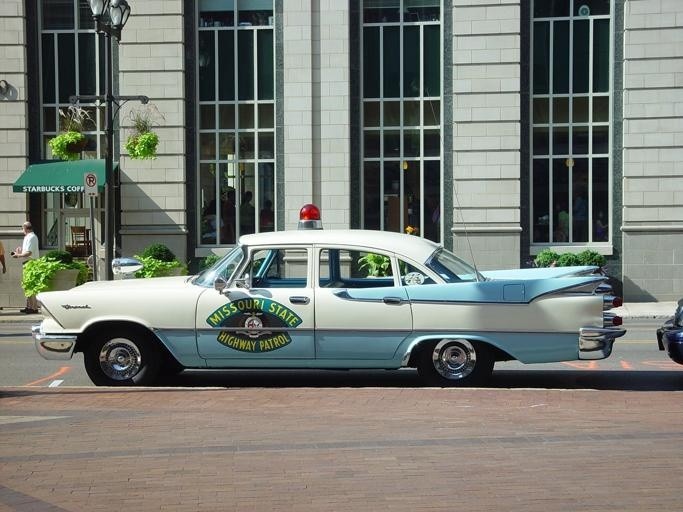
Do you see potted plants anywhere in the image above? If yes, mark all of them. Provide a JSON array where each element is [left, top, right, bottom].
[[48, 132, 87, 161], [21, 257, 93, 299], [133, 254, 189, 278]]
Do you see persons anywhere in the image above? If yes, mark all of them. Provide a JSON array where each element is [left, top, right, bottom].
[[0, 240, 7, 311], [9, 220, 40, 315], [221, 189, 235, 242], [260, 198, 273, 225], [553, 197, 568, 242], [203, 198, 224, 241], [239, 190, 254, 234], [592, 207, 607, 241]]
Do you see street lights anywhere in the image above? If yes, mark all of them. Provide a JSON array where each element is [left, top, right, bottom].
[[89, 0, 131, 280]]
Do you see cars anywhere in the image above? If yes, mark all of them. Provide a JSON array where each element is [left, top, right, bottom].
[[655, 298, 682, 366], [28, 205, 626, 385]]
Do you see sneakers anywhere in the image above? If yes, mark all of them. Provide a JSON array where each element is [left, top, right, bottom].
[[20, 307, 38, 314]]
